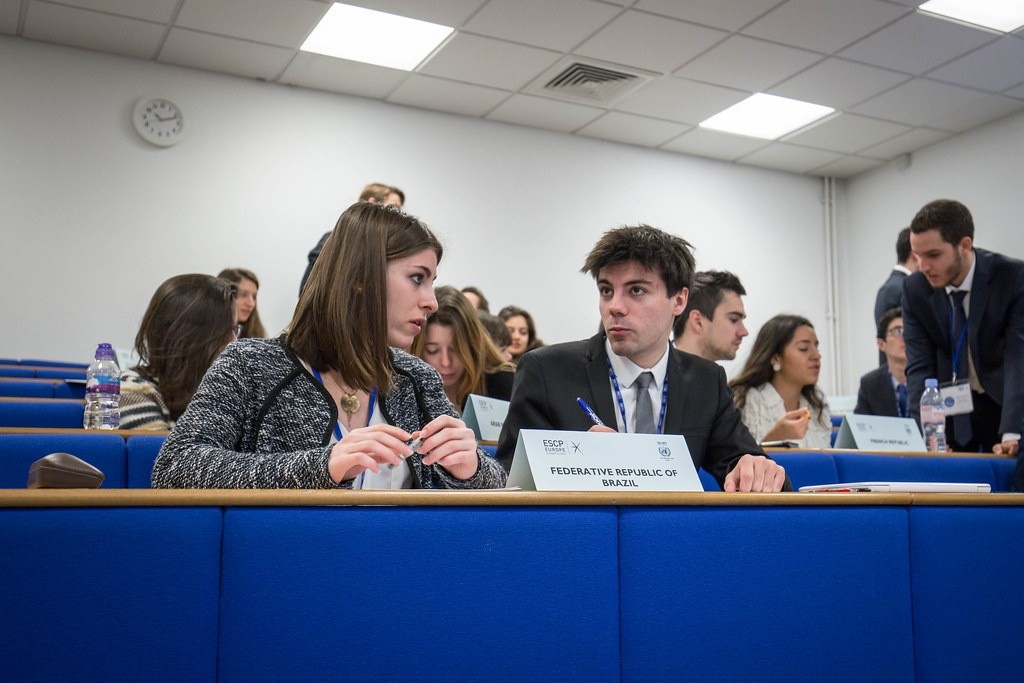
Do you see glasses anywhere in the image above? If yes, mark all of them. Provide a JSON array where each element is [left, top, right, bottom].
[[883, 327, 903, 338], [233, 325, 241, 337]]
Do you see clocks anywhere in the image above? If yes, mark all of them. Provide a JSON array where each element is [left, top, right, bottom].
[[132, 95, 188, 145]]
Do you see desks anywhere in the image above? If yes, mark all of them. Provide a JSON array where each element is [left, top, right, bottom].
[[0, 488, 1024, 683], [0, 356, 1016, 457]]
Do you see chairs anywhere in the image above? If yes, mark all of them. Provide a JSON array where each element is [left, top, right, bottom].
[[0, 435, 1024, 492]]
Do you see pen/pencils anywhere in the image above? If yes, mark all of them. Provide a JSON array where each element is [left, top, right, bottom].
[[809, 487, 872, 492], [576, 397, 605, 426], [388, 437, 424, 471]]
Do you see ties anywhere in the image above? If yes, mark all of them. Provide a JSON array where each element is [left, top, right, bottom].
[[897, 384, 907, 417], [950, 291, 972, 446], [633, 371, 656, 434]]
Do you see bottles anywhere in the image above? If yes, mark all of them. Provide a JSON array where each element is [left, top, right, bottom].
[[83, 342, 123, 431], [919, 378, 947, 452]]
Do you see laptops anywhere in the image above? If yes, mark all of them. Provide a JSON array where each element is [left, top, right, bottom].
[[799, 482, 991, 493]]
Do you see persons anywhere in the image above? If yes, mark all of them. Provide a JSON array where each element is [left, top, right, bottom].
[[118, 273, 242, 430], [902, 199, 1024, 456], [854, 309, 912, 417], [673, 273, 748, 362], [731, 315, 834, 448], [299, 183, 405, 293], [217, 269, 267, 338], [875, 229, 919, 367], [408, 288, 545, 417], [151, 200, 509, 489], [495, 227, 792, 493]]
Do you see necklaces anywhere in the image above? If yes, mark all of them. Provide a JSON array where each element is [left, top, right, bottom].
[[330, 371, 360, 425]]
